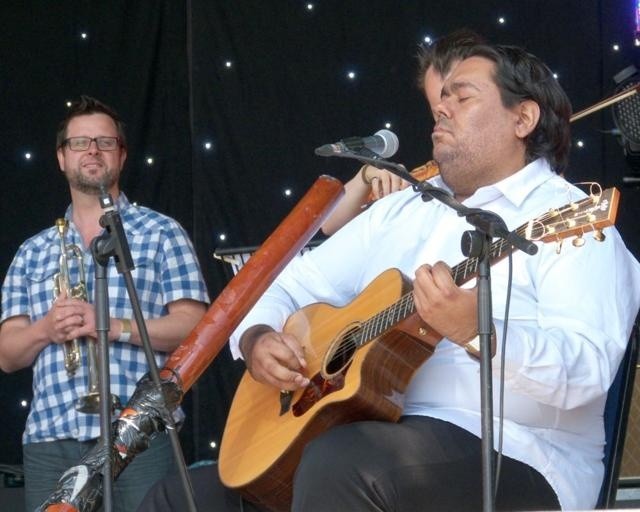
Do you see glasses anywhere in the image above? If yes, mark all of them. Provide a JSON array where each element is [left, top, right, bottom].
[[56, 136, 121, 151]]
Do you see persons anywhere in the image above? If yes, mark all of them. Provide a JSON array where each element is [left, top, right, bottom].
[[136, 31, 640, 510], [0, 95, 211, 512]]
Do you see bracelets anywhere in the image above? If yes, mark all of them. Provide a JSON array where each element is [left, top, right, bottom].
[[118, 318, 131, 342]]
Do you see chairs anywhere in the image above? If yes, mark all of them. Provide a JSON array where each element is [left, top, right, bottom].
[[596, 325, 640, 507]]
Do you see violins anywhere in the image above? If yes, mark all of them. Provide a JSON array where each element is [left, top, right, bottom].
[[370, 158, 440, 204]]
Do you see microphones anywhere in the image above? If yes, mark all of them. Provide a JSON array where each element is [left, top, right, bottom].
[[314, 130, 399, 158]]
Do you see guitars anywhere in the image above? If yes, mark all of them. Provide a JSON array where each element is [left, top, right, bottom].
[[217, 184, 620, 502]]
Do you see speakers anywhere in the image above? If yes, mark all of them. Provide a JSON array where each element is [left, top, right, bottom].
[[608, 64, 640, 154]]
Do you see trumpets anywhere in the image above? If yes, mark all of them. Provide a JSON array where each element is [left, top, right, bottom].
[[53, 216, 121, 412]]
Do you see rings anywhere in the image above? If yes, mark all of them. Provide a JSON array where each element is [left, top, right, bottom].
[[80, 315, 83, 326]]
